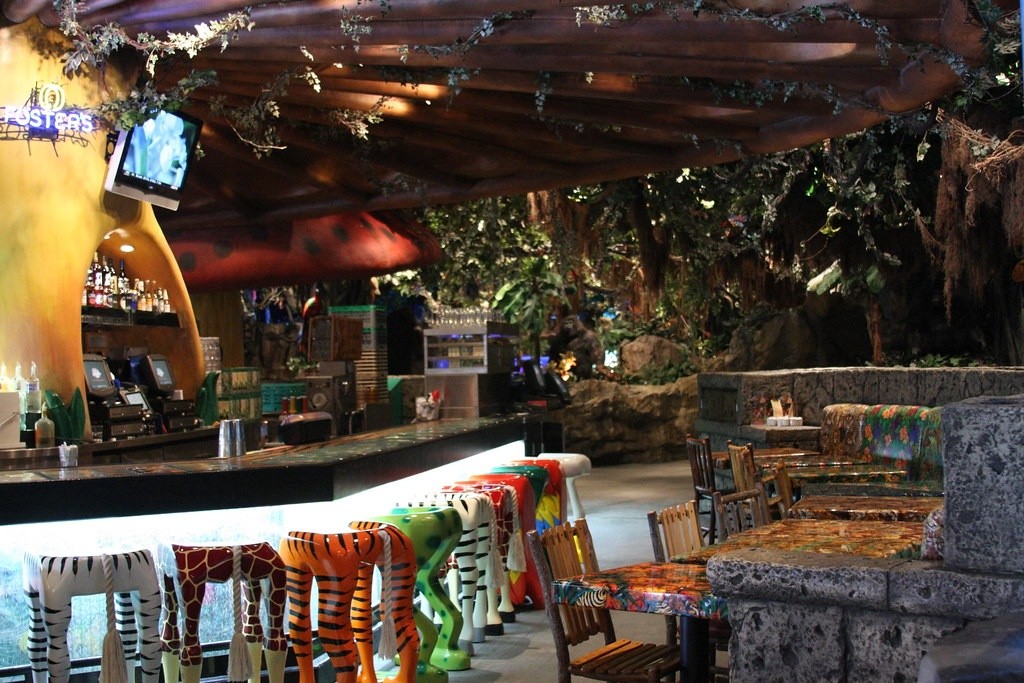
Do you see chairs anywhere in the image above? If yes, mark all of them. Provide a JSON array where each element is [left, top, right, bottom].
[[647, 499, 731, 683], [685, 432, 735, 546], [726, 444, 782, 532], [771, 456, 794, 511], [712, 481, 769, 544], [527, 518, 683, 683], [753, 466, 787, 524]]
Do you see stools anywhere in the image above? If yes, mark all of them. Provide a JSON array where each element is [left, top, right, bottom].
[[23, 453, 593, 683]]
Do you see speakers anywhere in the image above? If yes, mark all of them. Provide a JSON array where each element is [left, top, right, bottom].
[[308, 315, 363, 363]]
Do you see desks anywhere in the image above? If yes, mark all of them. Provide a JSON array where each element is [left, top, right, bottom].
[[553, 446, 944, 683], [742, 424, 820, 448]]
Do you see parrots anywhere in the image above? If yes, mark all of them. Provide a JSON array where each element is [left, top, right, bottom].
[[302, 283, 322, 320]]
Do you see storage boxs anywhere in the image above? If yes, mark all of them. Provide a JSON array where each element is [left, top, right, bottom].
[[329, 305, 388, 403], [261, 382, 304, 413]]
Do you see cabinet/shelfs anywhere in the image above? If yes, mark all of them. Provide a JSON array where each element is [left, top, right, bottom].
[[423, 320, 519, 375]]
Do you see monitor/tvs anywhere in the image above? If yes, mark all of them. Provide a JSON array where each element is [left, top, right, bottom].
[[522, 360, 544, 387], [119, 388, 153, 413], [146, 353, 177, 392], [84, 354, 115, 398], [103, 111, 203, 211], [543, 373, 570, 396]]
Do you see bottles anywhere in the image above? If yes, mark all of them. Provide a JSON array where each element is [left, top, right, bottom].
[[35, 403, 55, 448], [81, 252, 171, 313], [0, 363, 41, 431]]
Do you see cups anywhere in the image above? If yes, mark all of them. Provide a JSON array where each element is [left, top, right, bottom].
[[767, 417, 803, 427], [415, 398, 440, 421], [58, 446, 78, 467], [217, 419, 246, 458]]
